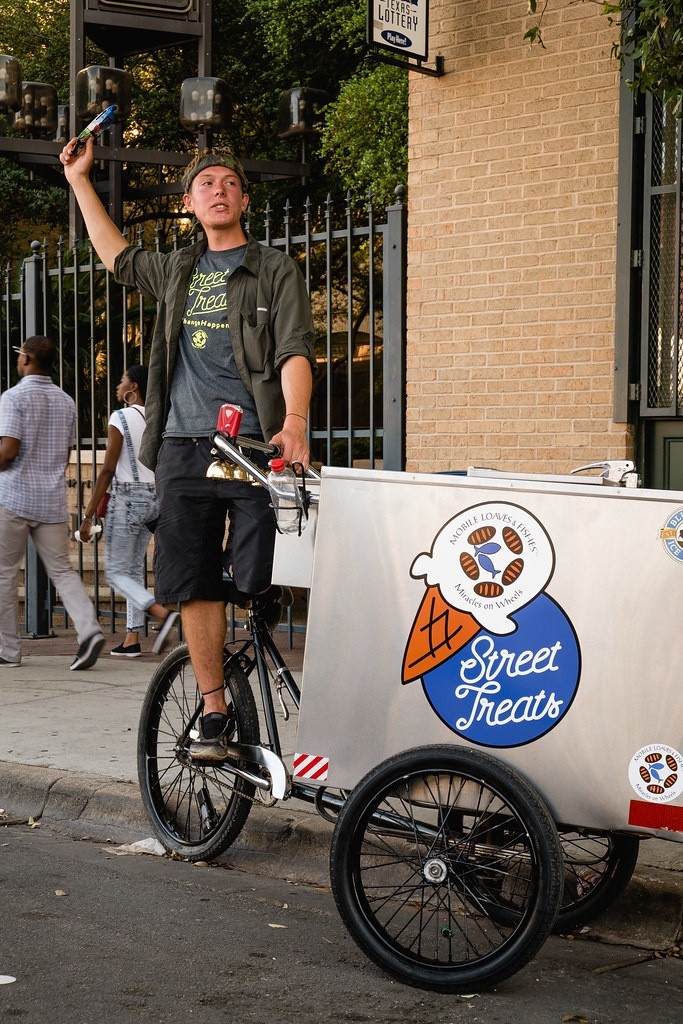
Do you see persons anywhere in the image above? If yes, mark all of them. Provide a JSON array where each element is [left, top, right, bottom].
[[79, 366, 180, 657], [0, 336, 106, 671], [57, 137, 319, 762]]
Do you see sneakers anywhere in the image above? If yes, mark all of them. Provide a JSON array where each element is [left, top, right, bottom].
[[69, 630, 107, 670], [0, 657, 20, 667], [190, 708, 234, 759], [261, 585, 286, 632], [110, 640, 143, 657], [151, 610, 181, 655]]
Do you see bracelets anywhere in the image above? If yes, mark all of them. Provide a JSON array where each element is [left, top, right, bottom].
[[284, 413, 306, 421], [82, 514, 92, 524]]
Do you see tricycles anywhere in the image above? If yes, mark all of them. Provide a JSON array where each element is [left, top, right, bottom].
[[133, 398, 654, 996]]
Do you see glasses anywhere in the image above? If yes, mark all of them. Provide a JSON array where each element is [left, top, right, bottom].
[[12, 345, 31, 361]]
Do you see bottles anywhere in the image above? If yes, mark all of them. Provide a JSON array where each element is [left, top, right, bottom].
[[267, 459, 307, 535]]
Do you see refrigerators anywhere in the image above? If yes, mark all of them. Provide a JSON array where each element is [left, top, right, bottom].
[[291, 464, 683, 843]]
